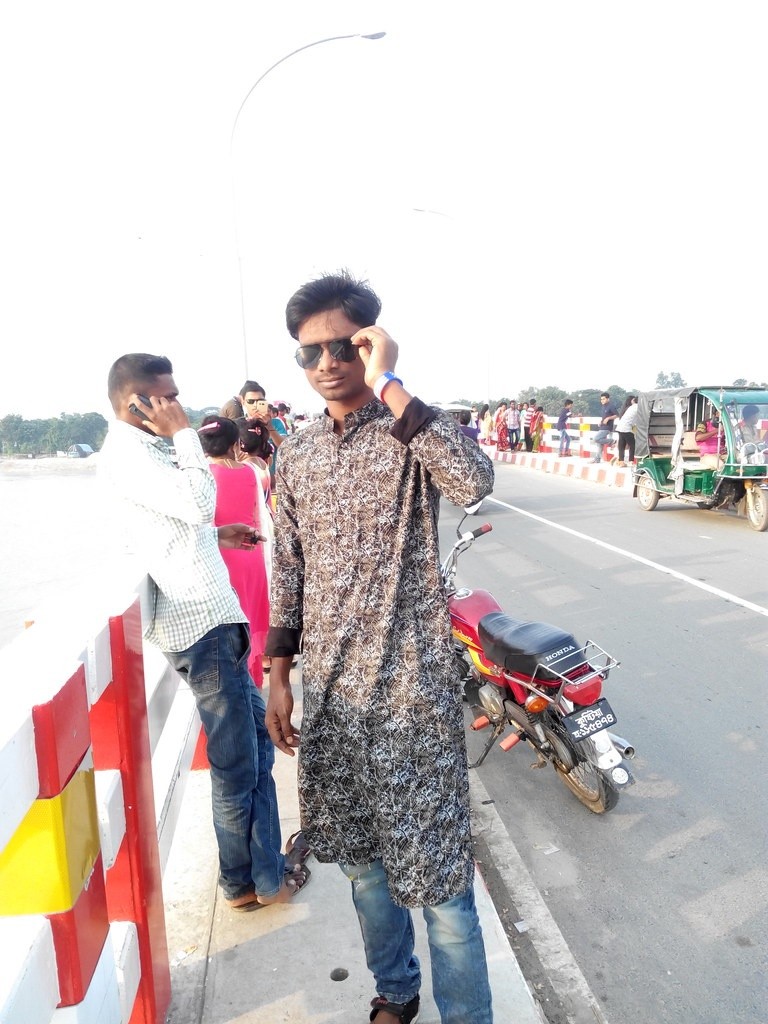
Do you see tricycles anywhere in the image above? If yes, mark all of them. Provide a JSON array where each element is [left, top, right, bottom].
[[631, 385, 768, 531]]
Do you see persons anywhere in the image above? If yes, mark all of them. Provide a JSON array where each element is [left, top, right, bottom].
[[558, 399, 574, 457], [590, 392, 619, 463], [694, 405, 768, 473], [263, 276, 493, 1024], [93, 353, 311, 911], [459, 392, 546, 453], [615, 396, 639, 468]]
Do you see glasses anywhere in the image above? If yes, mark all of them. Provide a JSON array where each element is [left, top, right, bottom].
[[295, 337, 362, 369], [246, 398, 265, 404]]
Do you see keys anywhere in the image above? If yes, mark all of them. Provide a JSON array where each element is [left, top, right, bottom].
[[250, 537, 258, 551]]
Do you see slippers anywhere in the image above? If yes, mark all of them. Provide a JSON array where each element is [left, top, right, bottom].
[[231, 864, 312, 912], [285, 830, 311, 865]]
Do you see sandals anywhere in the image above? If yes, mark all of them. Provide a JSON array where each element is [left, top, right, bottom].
[[370, 993, 420, 1024]]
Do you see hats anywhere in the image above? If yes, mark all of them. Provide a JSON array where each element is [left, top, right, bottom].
[[743, 405, 759, 418]]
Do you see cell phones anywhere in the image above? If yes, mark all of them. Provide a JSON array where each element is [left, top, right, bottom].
[[257, 400, 268, 415], [127, 394, 154, 426]]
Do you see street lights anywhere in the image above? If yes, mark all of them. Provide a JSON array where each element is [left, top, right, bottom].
[[222, 29, 388, 383]]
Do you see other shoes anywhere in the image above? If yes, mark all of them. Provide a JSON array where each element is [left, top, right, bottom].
[[558, 454, 572, 457], [590, 460, 599, 464], [620, 461, 627, 468]]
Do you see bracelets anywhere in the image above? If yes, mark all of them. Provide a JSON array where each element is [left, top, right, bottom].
[[373, 372, 403, 405]]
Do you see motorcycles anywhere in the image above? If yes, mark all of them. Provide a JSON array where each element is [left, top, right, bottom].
[[437, 499, 637, 814]]
